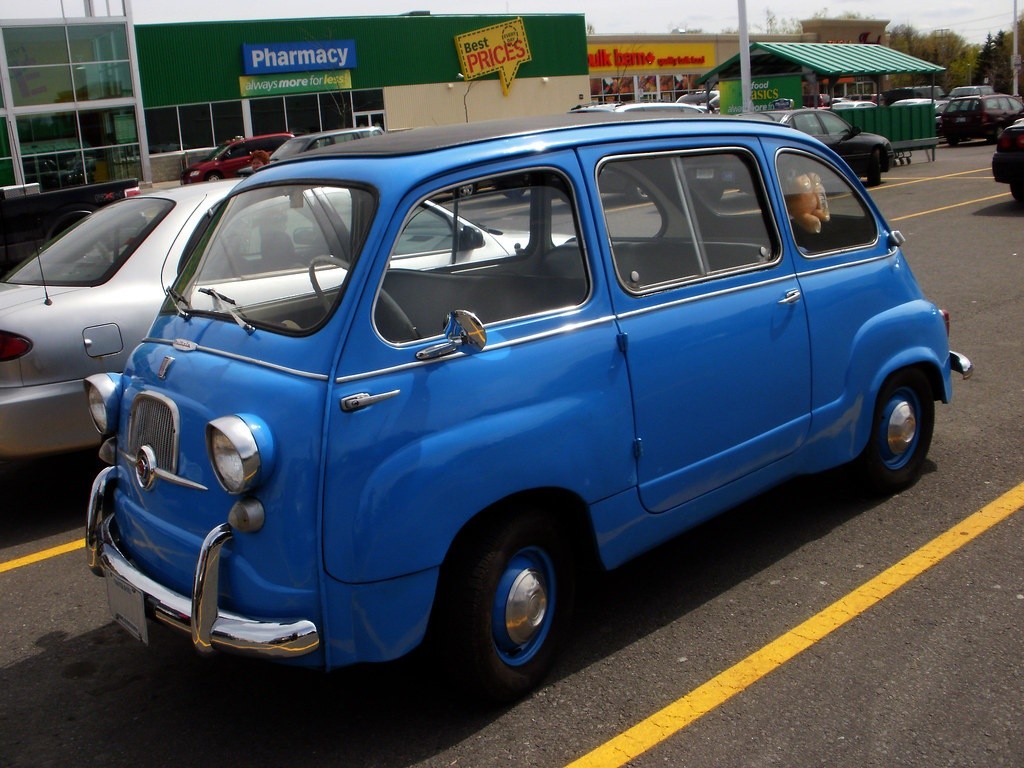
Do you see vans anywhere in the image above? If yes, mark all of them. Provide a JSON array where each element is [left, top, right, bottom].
[[82, 104, 976, 697]]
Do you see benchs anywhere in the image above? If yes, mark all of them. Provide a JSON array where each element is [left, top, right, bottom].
[[541, 237, 768, 304], [375, 268, 588, 343]]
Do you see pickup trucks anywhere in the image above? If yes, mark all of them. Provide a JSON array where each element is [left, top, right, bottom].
[[0, 177, 142, 249]]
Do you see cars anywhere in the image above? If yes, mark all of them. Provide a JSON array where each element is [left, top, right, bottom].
[[941, 93, 1024, 148], [235, 126, 386, 179], [0, 178, 581, 506], [712, 107, 895, 209], [992, 118, 1024, 203], [568, 85, 995, 135], [180, 133, 295, 186]]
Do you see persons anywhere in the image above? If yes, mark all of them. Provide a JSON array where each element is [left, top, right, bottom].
[[249, 150, 287, 256]]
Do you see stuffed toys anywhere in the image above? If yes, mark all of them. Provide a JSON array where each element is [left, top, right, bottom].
[[785, 169, 830, 234]]
